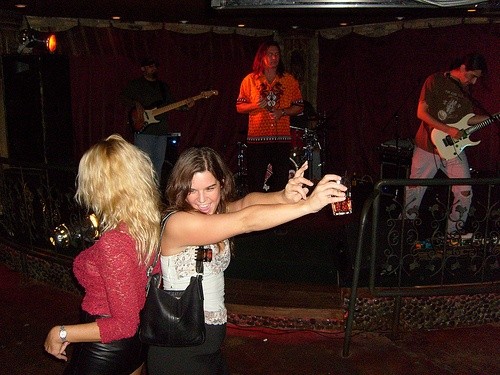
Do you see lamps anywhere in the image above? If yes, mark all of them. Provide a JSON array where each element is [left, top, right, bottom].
[[17, 16, 58, 55], [49, 215, 100, 248]]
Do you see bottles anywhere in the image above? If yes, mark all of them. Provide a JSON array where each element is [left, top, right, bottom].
[[416, 238, 460, 248]]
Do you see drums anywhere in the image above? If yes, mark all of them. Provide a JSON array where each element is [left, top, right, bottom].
[[289, 125, 306, 159], [263, 155, 302, 193]]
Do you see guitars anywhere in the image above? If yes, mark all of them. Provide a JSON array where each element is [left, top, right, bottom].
[[430, 111, 500, 160], [126, 88, 219, 134]]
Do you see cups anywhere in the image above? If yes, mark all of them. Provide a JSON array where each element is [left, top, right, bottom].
[[331, 176, 352, 216]]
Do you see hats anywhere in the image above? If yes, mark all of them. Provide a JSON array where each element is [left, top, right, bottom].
[[141, 58, 160, 66]]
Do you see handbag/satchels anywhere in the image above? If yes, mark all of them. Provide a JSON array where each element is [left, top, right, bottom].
[[138, 212, 207, 347]]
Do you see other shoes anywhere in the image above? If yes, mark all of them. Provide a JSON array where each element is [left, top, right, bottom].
[[274, 226, 289, 235], [447, 231, 473, 247]]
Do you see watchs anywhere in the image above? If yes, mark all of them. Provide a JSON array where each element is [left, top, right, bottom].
[[282, 109, 286, 116], [59, 325, 67, 342]]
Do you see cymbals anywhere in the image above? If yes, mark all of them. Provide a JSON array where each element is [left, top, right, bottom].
[[306, 116, 342, 121]]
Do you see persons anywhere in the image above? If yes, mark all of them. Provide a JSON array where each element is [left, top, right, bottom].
[[44, 133, 163, 375], [401, 53, 500, 250], [120, 57, 195, 209], [236, 39, 305, 194], [148, 146, 347, 375]]
